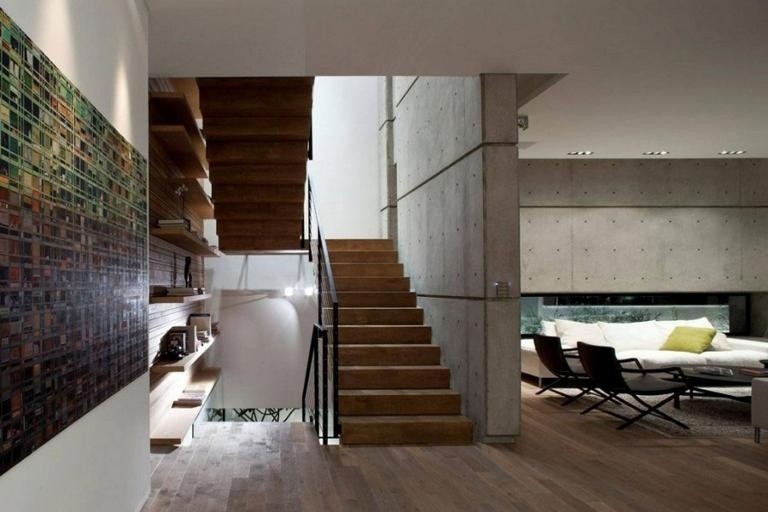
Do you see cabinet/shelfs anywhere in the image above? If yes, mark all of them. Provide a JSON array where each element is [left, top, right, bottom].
[[143, 93, 222, 446]]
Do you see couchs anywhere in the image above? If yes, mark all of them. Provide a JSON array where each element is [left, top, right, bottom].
[[520, 297, 768, 387]]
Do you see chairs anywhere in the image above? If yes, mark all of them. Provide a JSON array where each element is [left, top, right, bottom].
[[531, 333, 622, 406], [751, 378, 768, 443], [577, 341, 695, 430]]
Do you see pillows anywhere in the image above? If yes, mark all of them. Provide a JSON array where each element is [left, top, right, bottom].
[[539, 316, 731, 353]]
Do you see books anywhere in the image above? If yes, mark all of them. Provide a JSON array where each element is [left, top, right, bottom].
[[168, 287, 208, 296], [160, 219, 189, 229], [169, 312, 220, 353], [174, 389, 206, 407]]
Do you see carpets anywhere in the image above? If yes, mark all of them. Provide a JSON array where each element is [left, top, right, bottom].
[[546, 382, 768, 439]]
